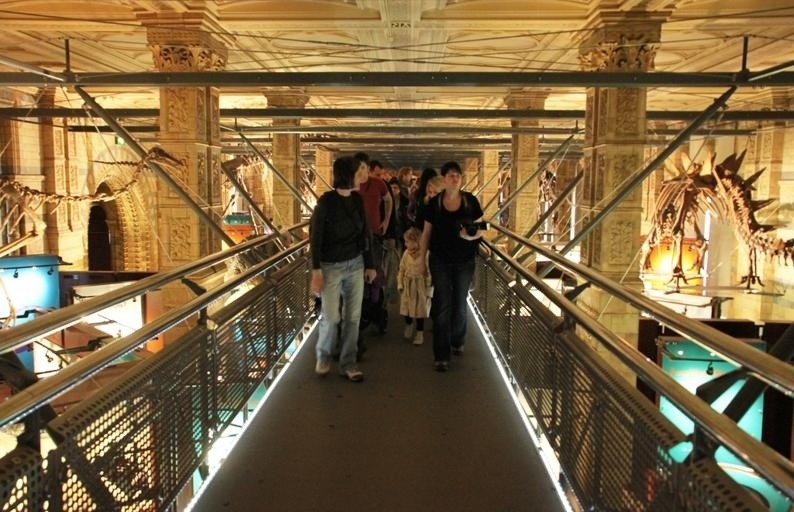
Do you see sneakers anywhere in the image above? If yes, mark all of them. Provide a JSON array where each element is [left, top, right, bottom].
[[314, 359, 363, 382], [432, 345, 464, 371], [404, 323, 424, 345]]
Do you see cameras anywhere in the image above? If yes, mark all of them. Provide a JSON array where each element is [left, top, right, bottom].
[[459, 216, 489, 235]]
